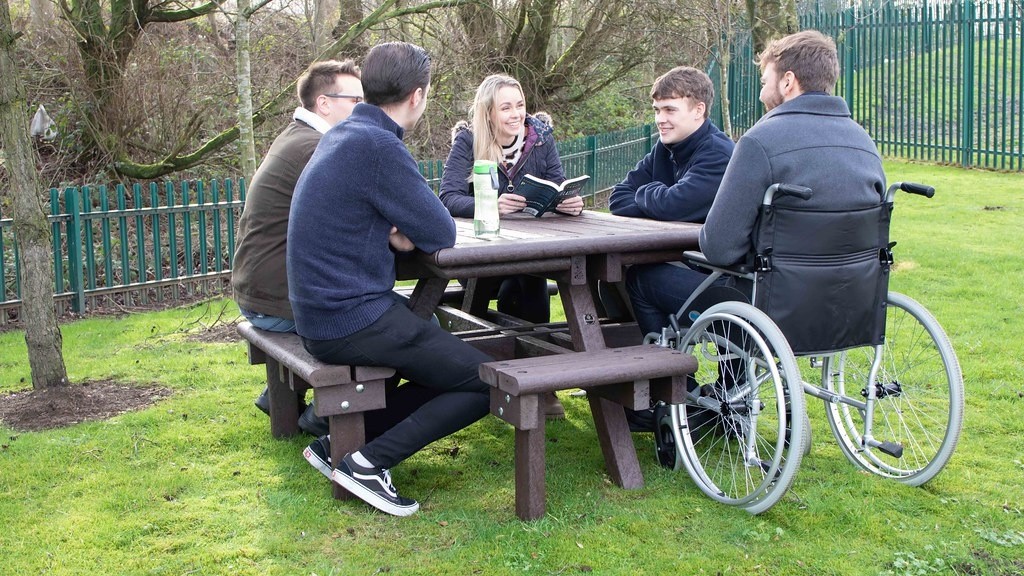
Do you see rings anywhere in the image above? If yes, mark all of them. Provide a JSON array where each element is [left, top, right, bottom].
[[574, 208, 576, 212]]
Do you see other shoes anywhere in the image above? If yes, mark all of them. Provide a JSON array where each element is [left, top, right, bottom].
[[544, 390, 566, 420], [253, 386, 308, 417], [624, 395, 704, 432], [296, 403, 329, 436]]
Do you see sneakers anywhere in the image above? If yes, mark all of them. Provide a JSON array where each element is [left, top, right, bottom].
[[302, 435, 333, 482], [330, 452, 419, 516]]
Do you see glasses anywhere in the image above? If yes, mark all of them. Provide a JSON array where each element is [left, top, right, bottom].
[[324, 92, 364, 104]]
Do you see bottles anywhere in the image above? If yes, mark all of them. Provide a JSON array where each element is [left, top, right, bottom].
[[473, 160, 500, 240]]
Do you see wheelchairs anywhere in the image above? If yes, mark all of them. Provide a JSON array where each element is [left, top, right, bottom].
[[643, 180, 966, 515]]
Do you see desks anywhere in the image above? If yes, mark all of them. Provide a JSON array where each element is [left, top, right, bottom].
[[394, 211, 705, 493]]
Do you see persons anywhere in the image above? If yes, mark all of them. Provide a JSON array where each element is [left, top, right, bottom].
[[625, 31, 886, 438], [438, 75, 585, 219], [287, 42, 498, 517], [231, 59, 443, 437], [598, 66, 736, 323]]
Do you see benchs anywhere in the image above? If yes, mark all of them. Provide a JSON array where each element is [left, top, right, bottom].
[[392, 281, 560, 310], [478, 344, 698, 522], [236, 320, 395, 500]]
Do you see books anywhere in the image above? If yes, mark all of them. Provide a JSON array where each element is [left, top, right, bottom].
[[511, 174, 591, 219]]
